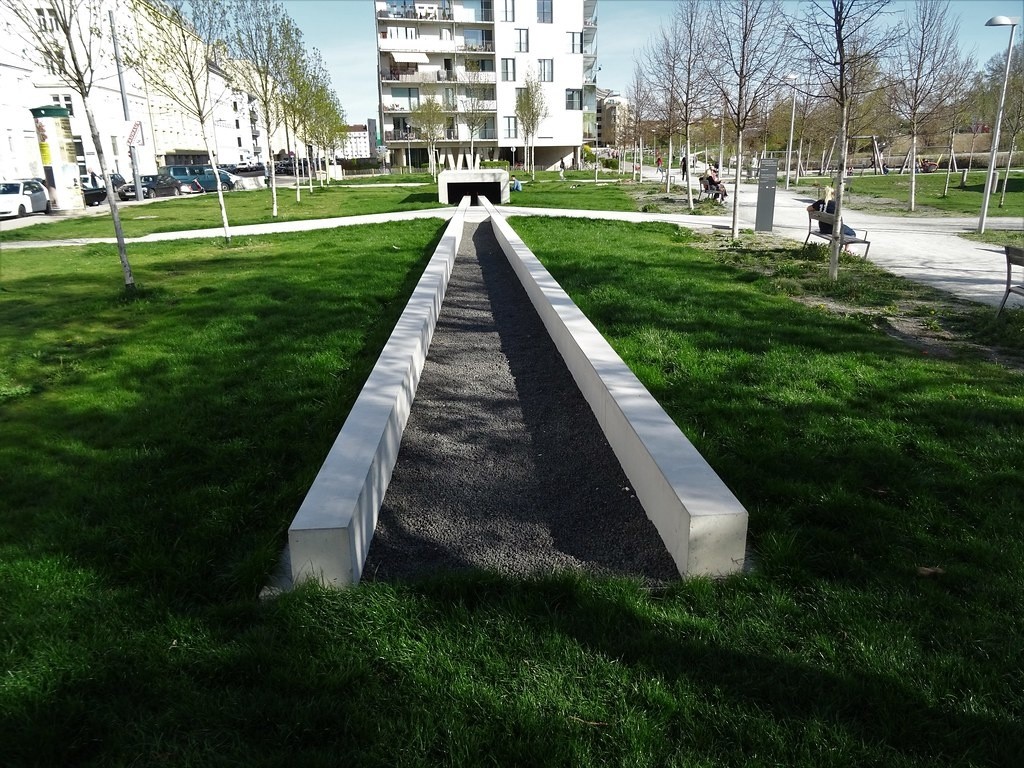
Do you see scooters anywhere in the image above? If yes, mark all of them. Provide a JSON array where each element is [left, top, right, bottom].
[[179, 173, 206, 195]]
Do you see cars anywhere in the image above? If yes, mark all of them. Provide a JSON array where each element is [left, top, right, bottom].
[[0, 178, 52, 220], [118, 174, 183, 202], [221, 159, 315, 176], [100, 173, 127, 193], [33, 169, 106, 213]]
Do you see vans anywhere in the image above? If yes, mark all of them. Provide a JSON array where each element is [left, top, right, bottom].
[[158, 163, 236, 192]]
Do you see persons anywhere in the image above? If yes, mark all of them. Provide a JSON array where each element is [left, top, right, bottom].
[[654, 152, 664, 174], [692, 153, 720, 180], [509, 174, 523, 192], [845, 157, 937, 176], [699, 167, 729, 201], [680, 152, 687, 181], [807, 185, 856, 256], [748, 156, 759, 180], [608, 147, 624, 160], [559, 156, 567, 181], [264, 160, 273, 188], [87, 167, 102, 188]]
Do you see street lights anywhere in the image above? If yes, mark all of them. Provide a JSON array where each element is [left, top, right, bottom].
[[977, 16, 1021, 234], [783, 73, 798, 190], [406, 124, 412, 174], [716, 103, 725, 179], [677, 127, 683, 161]]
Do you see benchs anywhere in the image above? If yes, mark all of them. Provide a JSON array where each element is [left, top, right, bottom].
[[661, 168, 675, 184], [803, 211, 871, 259], [994, 245, 1024, 318], [698, 180, 723, 204]]
